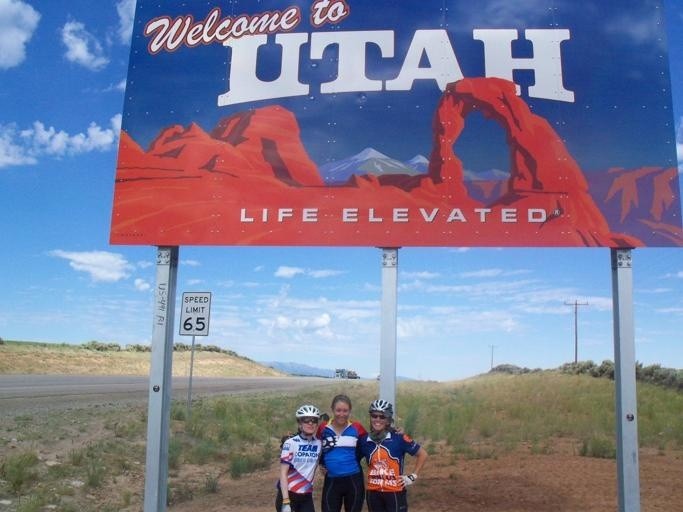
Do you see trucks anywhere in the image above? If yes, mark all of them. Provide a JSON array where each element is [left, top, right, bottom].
[[334, 368, 359, 380]]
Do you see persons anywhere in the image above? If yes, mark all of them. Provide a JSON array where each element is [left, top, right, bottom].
[[273, 404, 323, 511], [280, 395, 404, 511], [357, 398, 428, 511]]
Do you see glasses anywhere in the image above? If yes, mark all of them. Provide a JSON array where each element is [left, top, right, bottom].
[[369, 414, 385, 419], [303, 418, 318, 424]]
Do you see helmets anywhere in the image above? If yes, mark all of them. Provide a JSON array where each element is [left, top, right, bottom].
[[295, 405, 320, 420], [368, 400, 392, 418]]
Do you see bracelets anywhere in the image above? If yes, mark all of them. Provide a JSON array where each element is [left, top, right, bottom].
[[282, 498, 289, 502]]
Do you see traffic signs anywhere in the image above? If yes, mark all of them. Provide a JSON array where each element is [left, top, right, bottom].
[[179, 291, 212, 337]]
